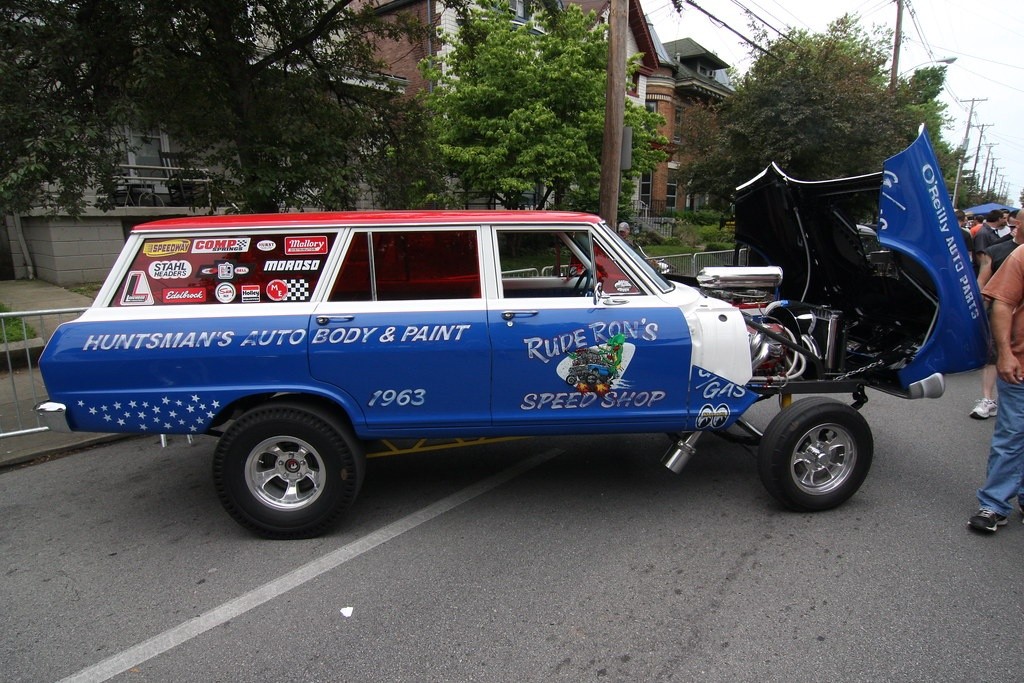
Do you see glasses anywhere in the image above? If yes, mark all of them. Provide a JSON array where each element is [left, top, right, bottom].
[[1007, 221, 1015, 228]]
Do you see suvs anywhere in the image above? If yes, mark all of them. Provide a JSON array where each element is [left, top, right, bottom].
[[35, 117, 999, 541]]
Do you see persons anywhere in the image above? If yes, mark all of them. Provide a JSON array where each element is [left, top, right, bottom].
[[618, 222, 632, 245], [968, 242, 1024, 532], [954, 208, 1020, 278], [969, 206, 1024, 420]]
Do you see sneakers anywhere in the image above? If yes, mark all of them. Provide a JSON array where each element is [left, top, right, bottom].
[[968, 507, 1007, 532], [1020, 507, 1024, 524], [970, 398, 998, 419]]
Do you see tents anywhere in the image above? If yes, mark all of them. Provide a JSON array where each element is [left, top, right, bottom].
[[962, 202, 1020, 218]]
